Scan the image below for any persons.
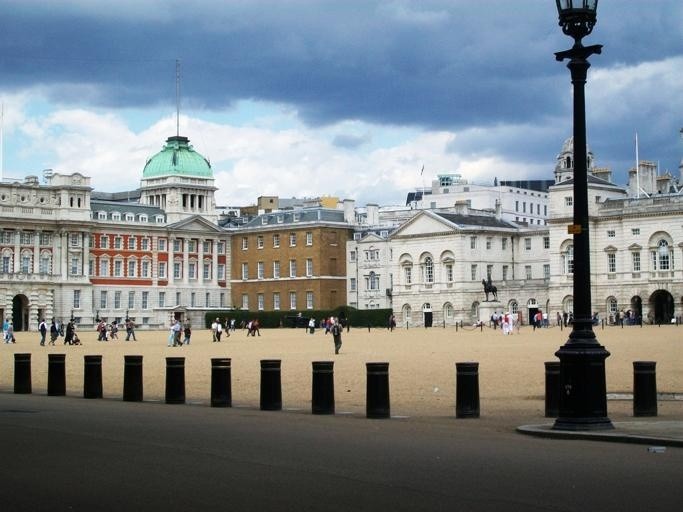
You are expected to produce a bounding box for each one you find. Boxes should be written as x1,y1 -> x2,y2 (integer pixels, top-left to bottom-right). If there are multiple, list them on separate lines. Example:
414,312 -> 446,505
492,312 -> 521,335
389,315 -> 395,330
533,311 -> 548,329
97,319 -> 137,341
477,320 -> 480,327
167,318 -> 192,347
557,310 -> 635,328
2,317 -> 75,346
211,317 -> 261,342
309,317 -> 345,354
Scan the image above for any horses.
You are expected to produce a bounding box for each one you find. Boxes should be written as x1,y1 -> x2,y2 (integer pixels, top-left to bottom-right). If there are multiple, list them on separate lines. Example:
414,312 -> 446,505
481,279 -> 498,301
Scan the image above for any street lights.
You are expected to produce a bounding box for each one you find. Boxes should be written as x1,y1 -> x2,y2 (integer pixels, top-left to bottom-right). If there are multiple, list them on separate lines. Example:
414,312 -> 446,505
551,0 -> 615,432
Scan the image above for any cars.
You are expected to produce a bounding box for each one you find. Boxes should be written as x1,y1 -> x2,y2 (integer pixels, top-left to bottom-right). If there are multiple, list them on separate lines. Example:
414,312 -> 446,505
282,316 -> 320,329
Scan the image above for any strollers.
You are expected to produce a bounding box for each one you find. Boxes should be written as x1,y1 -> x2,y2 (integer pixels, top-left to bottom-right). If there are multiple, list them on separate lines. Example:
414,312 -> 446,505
70,331 -> 82,346
